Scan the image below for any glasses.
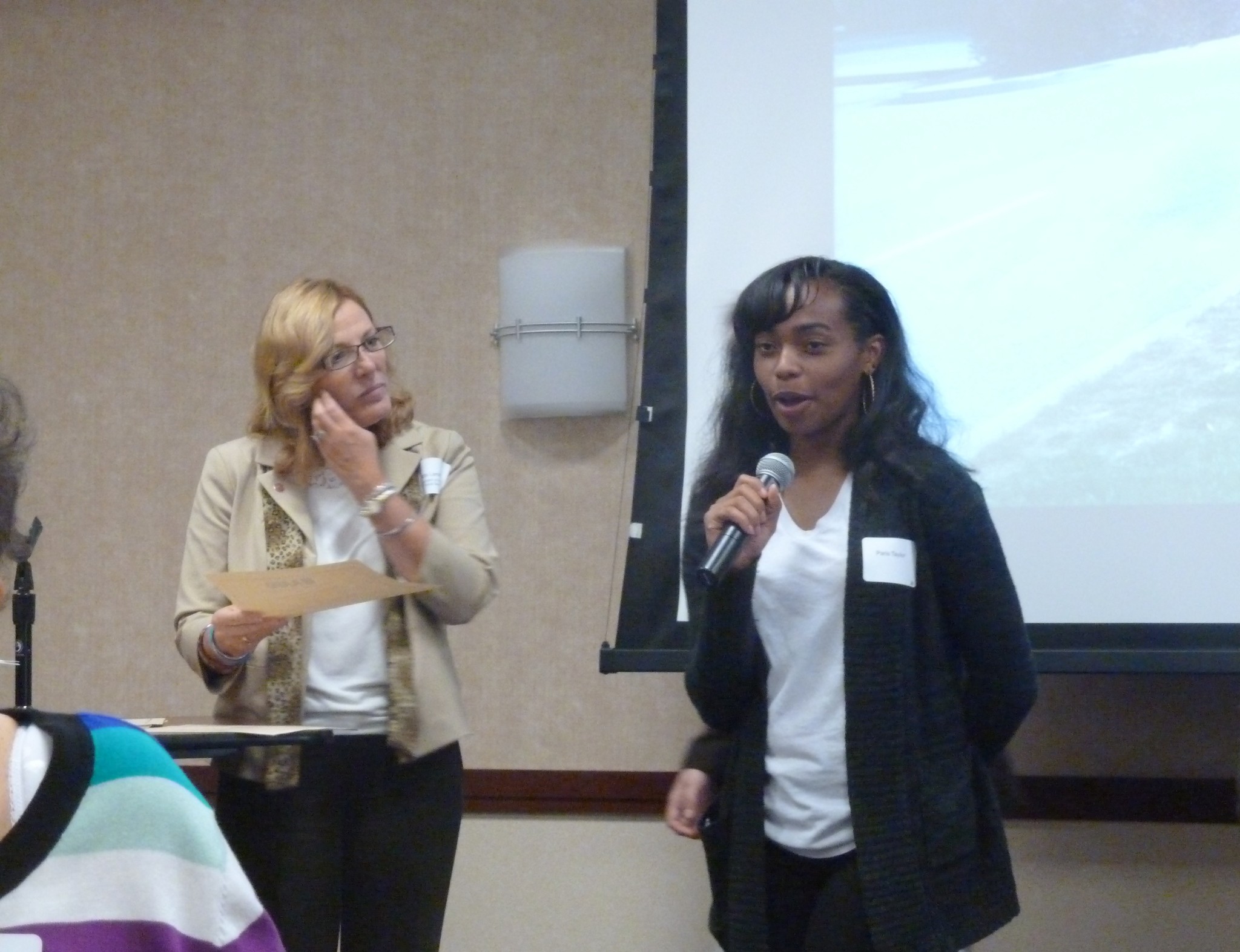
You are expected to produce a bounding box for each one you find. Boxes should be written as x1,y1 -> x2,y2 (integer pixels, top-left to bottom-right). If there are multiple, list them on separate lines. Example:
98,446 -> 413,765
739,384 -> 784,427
317,326 -> 395,371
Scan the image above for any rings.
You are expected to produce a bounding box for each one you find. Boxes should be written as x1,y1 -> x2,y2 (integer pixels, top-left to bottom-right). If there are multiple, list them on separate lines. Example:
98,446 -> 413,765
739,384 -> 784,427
311,427 -> 327,443
242,635 -> 252,642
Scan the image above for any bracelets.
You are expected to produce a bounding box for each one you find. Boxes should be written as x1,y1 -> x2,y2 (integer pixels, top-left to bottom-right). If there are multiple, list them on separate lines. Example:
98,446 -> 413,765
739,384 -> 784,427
203,624 -> 250,665
375,511 -> 420,538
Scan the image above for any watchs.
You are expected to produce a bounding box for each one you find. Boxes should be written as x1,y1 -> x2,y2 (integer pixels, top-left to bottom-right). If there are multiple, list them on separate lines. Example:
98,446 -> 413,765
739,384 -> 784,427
358,482 -> 398,517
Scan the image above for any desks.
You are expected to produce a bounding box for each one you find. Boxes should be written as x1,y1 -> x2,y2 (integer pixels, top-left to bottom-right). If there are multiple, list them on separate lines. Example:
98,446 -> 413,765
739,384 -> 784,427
128,726 -> 398,952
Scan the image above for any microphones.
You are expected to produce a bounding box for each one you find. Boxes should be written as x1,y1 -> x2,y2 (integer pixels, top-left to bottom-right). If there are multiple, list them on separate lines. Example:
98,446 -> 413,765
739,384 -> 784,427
695,452 -> 794,588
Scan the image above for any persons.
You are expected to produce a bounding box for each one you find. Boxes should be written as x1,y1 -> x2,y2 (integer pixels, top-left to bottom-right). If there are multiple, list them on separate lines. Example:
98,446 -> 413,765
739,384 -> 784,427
0,377 -> 287,952
172,276 -> 500,952
666,723 -> 718,838
681,256 -> 1037,952
